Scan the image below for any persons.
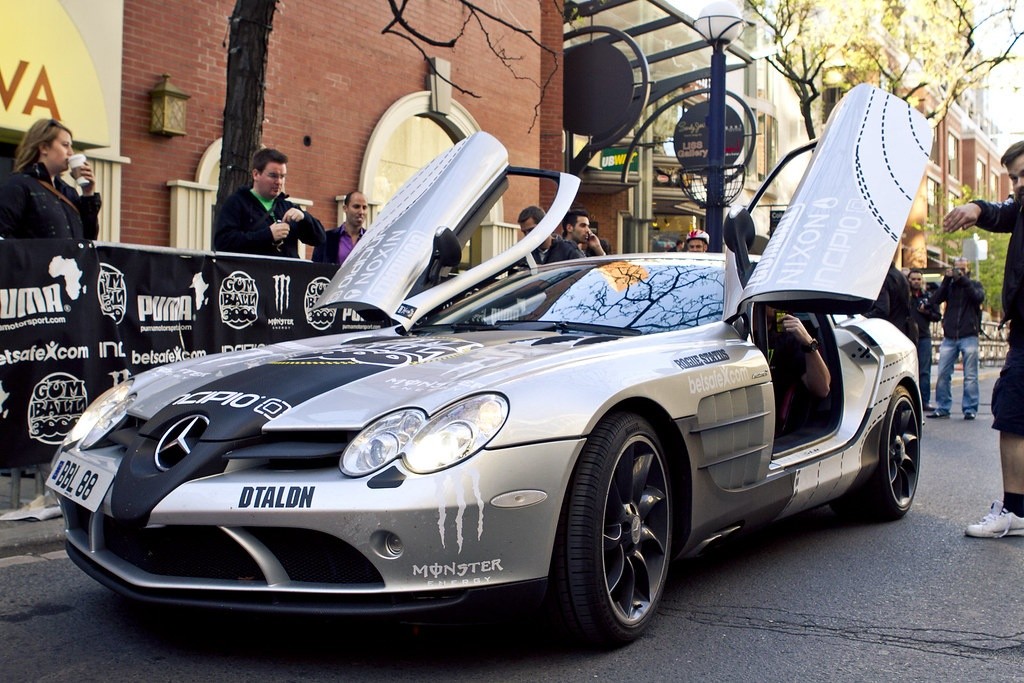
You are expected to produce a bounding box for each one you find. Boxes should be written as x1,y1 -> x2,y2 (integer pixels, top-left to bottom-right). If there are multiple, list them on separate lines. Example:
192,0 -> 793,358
560,210 -> 609,257
685,229 -> 711,251
0,119 -> 103,243
312,192 -> 367,264
667,240 -> 685,252
942,140 -> 1024,538
907,270 -> 943,410
765,305 -> 830,399
212,147 -> 327,258
514,205 -> 587,272
920,257 -> 985,420
863,247 -> 919,345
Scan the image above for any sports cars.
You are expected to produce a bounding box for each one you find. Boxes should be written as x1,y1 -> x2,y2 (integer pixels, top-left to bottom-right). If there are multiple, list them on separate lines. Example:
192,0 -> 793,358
42,83 -> 936,649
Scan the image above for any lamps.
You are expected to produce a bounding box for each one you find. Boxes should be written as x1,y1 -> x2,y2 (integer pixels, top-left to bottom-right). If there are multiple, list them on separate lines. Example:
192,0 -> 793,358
149,72 -> 189,136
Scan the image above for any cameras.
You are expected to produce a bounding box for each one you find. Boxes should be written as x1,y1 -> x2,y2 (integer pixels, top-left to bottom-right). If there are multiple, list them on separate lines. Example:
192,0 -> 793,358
952,267 -> 965,275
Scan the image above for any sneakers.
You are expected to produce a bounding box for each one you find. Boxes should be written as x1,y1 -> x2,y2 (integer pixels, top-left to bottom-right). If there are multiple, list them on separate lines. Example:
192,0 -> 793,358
965,500 -> 1024,538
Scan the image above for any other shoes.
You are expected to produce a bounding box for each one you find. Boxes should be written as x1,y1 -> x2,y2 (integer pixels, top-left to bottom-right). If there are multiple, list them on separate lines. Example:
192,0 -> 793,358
965,412 -> 974,419
926,412 -> 950,419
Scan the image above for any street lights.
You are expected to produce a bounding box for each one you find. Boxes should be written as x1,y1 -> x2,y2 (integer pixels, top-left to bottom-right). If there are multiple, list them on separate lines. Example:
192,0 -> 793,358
694,1 -> 749,252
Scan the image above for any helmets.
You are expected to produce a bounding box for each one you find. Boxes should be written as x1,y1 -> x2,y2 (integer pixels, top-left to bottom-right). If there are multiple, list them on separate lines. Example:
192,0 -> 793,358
686,229 -> 710,245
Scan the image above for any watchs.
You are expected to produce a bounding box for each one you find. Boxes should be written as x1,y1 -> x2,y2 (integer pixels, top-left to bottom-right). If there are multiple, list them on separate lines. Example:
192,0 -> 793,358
804,338 -> 818,353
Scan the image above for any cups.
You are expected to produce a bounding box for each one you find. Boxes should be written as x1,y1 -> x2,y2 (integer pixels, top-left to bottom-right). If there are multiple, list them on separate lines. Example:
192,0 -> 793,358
67,154 -> 89,186
